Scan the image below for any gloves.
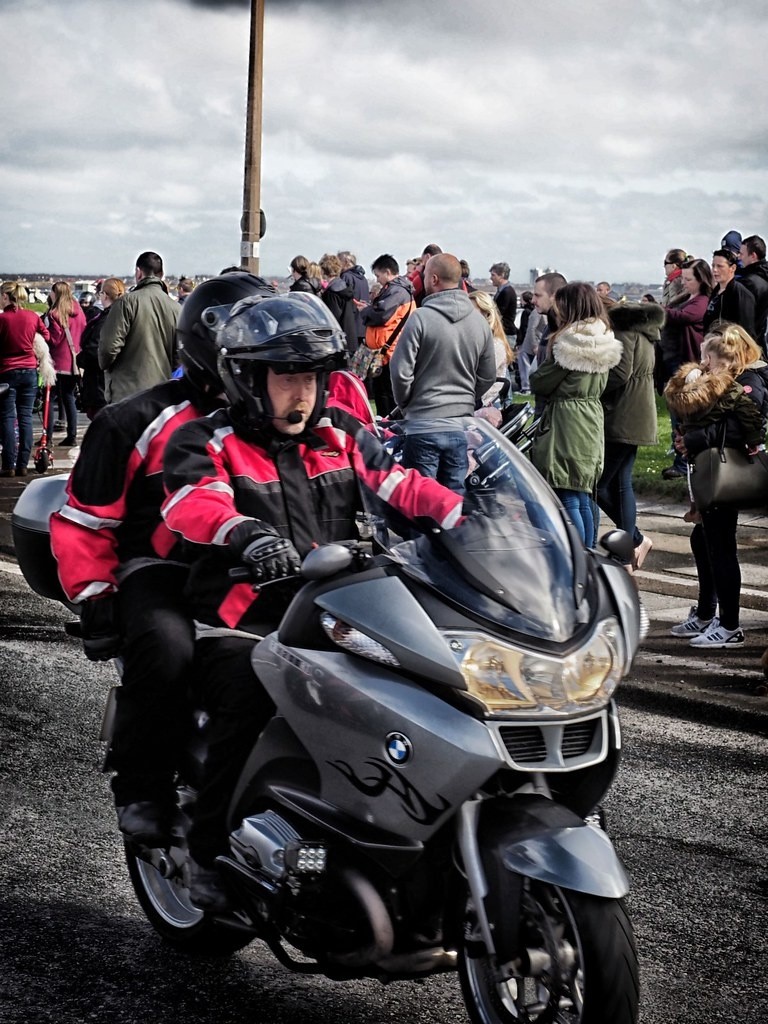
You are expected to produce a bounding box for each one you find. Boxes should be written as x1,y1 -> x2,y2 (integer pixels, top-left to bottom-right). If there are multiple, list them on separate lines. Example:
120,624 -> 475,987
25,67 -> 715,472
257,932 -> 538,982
228,520 -> 303,583
81,594 -> 133,663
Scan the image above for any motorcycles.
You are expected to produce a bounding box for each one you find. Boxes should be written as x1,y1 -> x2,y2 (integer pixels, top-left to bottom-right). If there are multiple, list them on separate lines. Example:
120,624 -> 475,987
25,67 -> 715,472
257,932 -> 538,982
12,409 -> 646,1024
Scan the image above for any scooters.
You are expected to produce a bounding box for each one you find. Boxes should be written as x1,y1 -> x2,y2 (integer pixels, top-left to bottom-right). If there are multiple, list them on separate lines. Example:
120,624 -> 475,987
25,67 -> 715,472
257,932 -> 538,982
32,366 -> 56,475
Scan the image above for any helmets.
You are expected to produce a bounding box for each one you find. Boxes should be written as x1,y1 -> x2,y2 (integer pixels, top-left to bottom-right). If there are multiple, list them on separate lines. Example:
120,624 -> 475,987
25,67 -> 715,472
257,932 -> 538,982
176,270 -> 281,397
218,292 -> 350,430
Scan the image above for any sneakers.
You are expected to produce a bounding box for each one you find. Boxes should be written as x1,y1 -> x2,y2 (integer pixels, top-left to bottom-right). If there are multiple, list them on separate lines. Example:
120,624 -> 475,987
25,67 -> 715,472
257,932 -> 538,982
671,605 -> 716,637
689,616 -> 744,648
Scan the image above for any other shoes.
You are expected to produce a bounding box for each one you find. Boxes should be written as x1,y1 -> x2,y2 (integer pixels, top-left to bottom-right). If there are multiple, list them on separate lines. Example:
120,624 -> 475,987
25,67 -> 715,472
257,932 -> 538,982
661,465 -> 686,479
15,467 -> 27,476
35,438 -> 52,446
631,537 -> 654,568
182,856 -> 226,910
58,434 -> 77,447
117,801 -> 175,839
0,469 -> 16,478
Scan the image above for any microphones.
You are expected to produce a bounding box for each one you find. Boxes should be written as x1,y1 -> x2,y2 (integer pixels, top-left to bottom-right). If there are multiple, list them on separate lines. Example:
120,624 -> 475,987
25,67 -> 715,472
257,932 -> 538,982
259,412 -> 303,424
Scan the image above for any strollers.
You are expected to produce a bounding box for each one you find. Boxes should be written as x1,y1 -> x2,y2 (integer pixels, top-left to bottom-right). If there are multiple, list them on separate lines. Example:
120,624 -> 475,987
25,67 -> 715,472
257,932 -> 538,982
328,369 -> 550,491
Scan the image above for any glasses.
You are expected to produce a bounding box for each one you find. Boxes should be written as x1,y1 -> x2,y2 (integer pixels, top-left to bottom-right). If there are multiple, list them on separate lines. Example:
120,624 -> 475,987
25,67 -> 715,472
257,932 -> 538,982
664,259 -> 679,266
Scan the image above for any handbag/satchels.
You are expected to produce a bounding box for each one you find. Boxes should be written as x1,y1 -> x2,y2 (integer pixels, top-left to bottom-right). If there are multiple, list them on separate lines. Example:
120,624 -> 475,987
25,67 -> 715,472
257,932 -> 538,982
350,343 -> 383,382
73,352 -> 83,375
689,423 -> 768,514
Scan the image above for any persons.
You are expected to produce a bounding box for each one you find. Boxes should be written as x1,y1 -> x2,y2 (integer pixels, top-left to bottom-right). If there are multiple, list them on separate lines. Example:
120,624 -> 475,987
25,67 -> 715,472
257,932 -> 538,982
51,268 -> 276,848
0,229 -> 768,650
153,292 -> 475,867
